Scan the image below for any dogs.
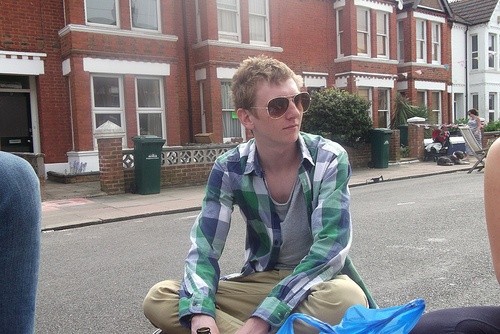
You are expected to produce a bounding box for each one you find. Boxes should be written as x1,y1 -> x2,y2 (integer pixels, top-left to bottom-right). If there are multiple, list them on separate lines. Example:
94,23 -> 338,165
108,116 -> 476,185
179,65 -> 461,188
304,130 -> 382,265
436,150 -> 470,166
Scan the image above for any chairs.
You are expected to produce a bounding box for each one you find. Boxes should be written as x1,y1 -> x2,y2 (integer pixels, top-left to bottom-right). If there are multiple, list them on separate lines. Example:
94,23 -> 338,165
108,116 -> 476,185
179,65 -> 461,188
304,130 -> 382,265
459,126 -> 489,174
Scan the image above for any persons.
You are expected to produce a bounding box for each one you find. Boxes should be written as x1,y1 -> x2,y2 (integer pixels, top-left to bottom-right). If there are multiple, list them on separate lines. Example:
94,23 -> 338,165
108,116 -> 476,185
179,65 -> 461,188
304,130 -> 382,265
143,56 -> 380,334
432,126 -> 452,156
462,109 -> 481,161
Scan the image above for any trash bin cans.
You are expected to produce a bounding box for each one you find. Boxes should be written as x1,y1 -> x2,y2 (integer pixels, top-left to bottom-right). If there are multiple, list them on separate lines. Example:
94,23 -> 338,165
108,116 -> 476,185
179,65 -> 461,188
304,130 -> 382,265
367,128 -> 392,168
130,135 -> 166,194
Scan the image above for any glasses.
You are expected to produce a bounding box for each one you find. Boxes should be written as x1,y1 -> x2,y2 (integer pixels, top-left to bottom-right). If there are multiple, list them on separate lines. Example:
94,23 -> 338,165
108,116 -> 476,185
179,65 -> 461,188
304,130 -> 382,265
247,92 -> 311,119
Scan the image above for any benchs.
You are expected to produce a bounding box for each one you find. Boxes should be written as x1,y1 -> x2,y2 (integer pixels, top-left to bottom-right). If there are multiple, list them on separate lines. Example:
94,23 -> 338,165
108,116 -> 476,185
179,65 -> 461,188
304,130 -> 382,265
424,146 -> 449,162
